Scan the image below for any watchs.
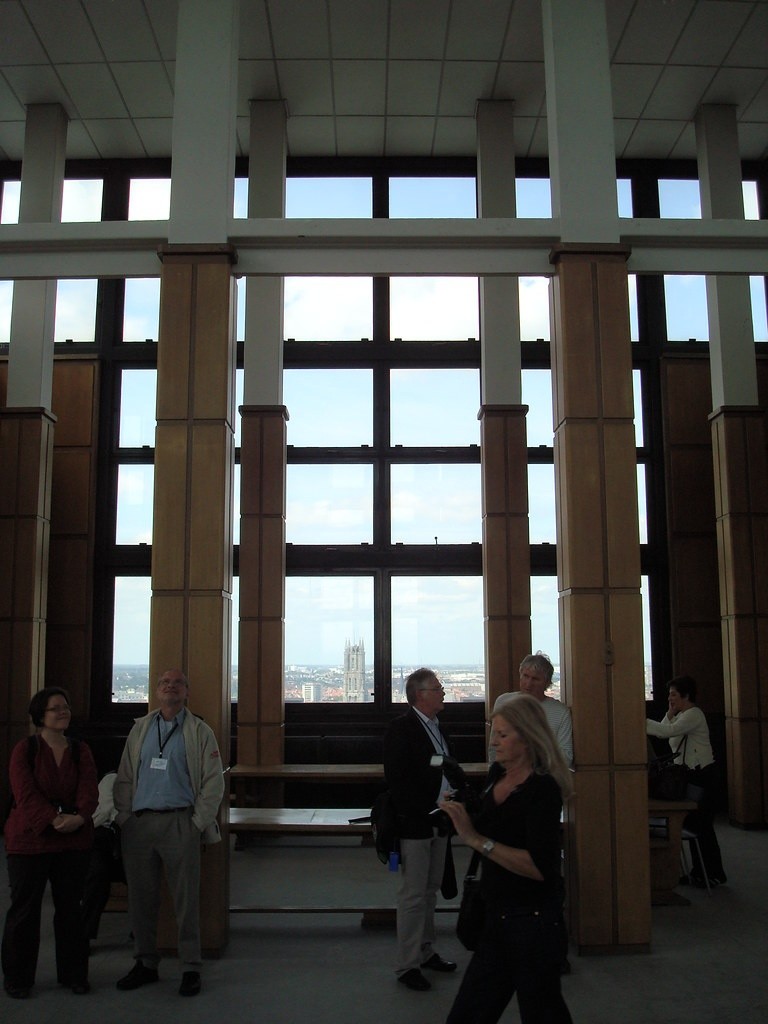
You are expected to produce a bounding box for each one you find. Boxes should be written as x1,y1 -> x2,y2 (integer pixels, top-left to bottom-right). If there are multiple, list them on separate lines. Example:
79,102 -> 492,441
482,840 -> 496,856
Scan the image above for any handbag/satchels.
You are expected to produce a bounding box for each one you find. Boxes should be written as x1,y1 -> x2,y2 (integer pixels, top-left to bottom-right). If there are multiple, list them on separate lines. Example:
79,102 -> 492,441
441,836 -> 458,900
456,877 -> 489,951
648,763 -> 690,801
370,791 -> 402,871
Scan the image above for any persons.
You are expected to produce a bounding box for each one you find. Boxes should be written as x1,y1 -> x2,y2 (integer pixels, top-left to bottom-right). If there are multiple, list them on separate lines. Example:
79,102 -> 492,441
647,677 -> 727,888
383,669 -> 467,991
488,655 -> 572,975
0,686 -> 99,999
80,773 -> 135,952
439,694 -> 574,1024
113,671 -> 225,995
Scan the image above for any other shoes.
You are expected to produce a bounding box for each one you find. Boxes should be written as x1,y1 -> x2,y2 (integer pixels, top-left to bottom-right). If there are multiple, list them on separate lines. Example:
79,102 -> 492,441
178,971 -> 200,996
560,958 -> 572,974
420,953 -> 457,972
116,960 -> 158,990
70,982 -> 91,995
679,874 -> 727,889
398,969 -> 431,991
3,981 -> 30,999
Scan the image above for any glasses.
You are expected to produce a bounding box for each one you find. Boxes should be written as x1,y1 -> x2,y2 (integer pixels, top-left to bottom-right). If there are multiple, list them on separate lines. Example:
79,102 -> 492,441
419,687 -> 444,694
157,680 -> 188,687
45,704 -> 71,713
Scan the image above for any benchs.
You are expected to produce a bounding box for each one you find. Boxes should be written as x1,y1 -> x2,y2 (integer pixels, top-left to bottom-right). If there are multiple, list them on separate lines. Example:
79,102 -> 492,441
228,806 -> 564,931
230,763 -> 493,852
648,799 -> 700,906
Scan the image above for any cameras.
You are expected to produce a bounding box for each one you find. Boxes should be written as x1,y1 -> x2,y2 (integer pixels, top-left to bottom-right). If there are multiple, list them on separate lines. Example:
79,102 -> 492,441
429,753 -> 489,840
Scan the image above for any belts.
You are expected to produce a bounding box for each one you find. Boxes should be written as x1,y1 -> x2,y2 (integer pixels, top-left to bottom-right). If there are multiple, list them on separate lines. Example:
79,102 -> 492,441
132,807 -> 187,817
